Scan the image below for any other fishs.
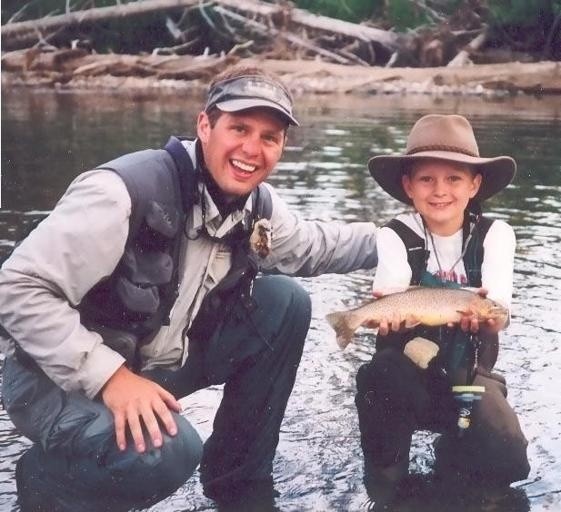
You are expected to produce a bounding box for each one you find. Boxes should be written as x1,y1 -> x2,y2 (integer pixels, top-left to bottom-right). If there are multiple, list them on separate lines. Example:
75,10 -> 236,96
324,286 -> 510,351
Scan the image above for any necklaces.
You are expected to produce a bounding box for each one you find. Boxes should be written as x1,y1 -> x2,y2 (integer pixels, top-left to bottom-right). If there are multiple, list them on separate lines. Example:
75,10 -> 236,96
419,212 -> 479,288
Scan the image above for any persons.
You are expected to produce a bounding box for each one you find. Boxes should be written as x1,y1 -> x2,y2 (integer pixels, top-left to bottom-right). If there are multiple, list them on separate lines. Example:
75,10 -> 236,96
355,115 -> 530,512
0,69 -> 377,512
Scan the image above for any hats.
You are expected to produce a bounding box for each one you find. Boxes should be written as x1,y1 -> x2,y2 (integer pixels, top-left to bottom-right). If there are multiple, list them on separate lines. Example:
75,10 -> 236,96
204,75 -> 300,126
368,113 -> 518,206
